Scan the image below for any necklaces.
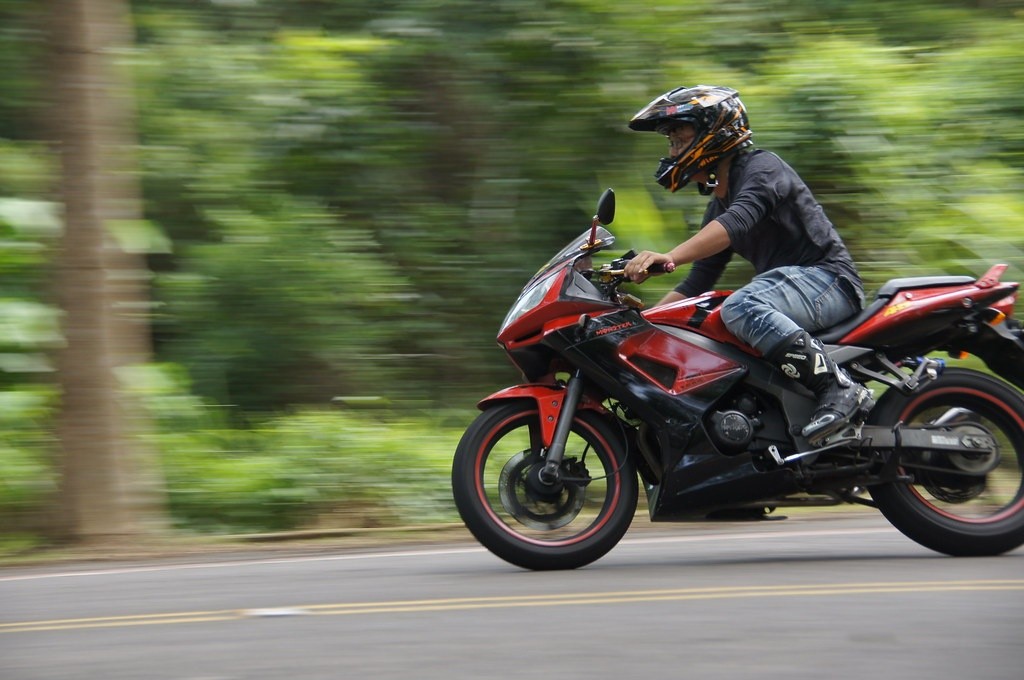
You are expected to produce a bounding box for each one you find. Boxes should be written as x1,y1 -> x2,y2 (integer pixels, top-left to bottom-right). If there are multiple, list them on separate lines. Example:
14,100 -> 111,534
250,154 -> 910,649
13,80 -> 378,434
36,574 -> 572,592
714,171 -> 730,210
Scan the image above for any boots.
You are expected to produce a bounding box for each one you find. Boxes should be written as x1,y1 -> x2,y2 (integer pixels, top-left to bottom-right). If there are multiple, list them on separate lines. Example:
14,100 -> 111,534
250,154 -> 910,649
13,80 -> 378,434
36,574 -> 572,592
761,328 -> 870,445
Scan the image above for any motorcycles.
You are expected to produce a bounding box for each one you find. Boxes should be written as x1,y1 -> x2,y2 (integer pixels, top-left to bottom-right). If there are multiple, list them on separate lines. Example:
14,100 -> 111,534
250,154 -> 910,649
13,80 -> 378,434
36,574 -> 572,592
450,188 -> 1024,574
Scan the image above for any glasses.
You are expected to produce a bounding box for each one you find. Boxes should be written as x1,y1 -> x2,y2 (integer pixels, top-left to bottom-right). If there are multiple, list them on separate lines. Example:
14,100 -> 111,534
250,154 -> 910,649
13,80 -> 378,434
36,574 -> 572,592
663,133 -> 695,149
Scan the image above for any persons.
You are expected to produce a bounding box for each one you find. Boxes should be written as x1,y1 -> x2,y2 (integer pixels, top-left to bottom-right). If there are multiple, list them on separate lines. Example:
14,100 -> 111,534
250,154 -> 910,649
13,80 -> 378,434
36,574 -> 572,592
624,86 -> 868,438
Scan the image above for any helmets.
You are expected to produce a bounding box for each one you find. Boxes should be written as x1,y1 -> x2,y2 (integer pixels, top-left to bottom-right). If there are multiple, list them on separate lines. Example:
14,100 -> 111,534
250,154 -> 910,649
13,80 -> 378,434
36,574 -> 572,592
628,85 -> 753,194
